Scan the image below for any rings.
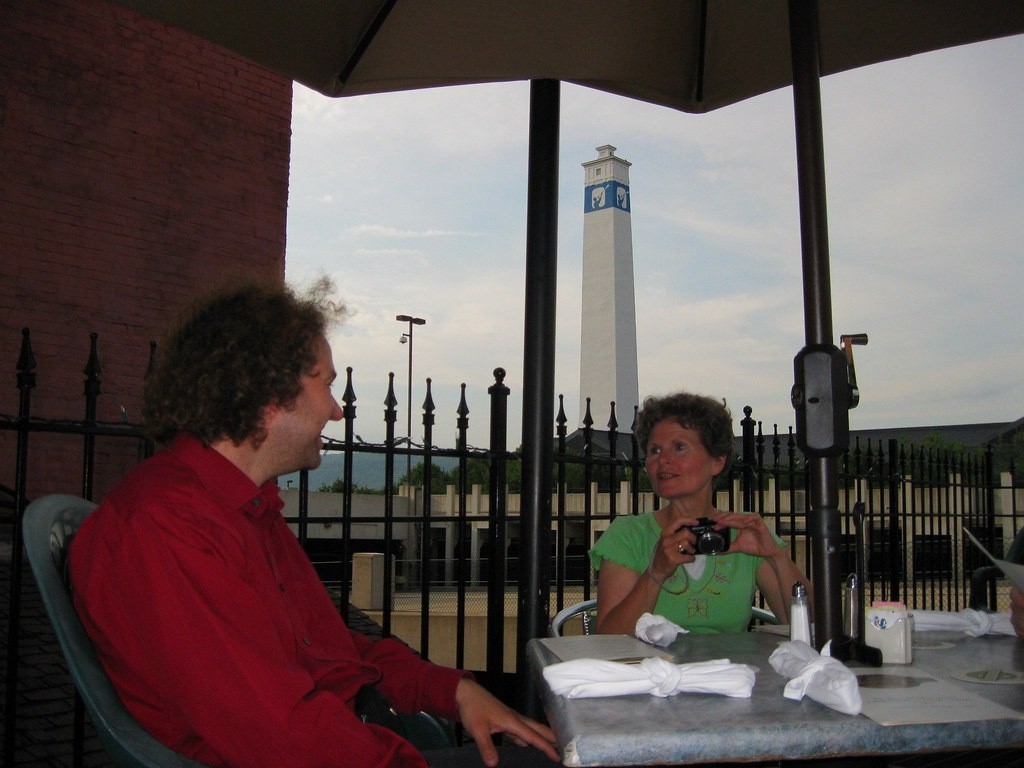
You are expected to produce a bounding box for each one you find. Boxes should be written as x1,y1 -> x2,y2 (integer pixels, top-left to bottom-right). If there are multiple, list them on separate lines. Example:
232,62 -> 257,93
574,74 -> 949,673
675,541 -> 686,553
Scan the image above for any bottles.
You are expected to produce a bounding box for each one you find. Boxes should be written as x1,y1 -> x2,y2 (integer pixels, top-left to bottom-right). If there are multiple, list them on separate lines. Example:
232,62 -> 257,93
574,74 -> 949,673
789,580 -> 811,647
843,571 -> 859,639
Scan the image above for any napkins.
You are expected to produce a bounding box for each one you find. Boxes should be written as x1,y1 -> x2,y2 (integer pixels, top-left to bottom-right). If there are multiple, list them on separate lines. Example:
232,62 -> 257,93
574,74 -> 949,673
633,612 -> 690,647
542,655 -> 756,701
767,638 -> 863,717
910,608 -> 1016,637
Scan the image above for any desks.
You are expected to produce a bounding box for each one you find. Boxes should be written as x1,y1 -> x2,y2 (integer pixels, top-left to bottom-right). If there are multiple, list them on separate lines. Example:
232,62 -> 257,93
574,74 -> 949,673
525,631 -> 1024,768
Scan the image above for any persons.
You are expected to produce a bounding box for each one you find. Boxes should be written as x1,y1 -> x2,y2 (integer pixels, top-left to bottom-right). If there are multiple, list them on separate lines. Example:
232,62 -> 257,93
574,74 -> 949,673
587,393 -> 814,636
67,280 -> 561,768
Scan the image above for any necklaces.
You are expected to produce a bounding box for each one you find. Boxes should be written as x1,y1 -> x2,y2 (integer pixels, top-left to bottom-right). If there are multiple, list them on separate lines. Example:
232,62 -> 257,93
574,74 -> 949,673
676,555 -> 717,594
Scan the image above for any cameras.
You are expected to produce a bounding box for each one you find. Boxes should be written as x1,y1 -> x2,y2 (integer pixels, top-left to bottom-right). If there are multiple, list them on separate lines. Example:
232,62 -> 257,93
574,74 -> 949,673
677,518 -> 730,556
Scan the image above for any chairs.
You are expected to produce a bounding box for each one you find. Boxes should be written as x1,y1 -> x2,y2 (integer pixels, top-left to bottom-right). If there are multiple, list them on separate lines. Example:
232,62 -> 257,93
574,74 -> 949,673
548,599 -> 780,638
22,493 -> 462,768
